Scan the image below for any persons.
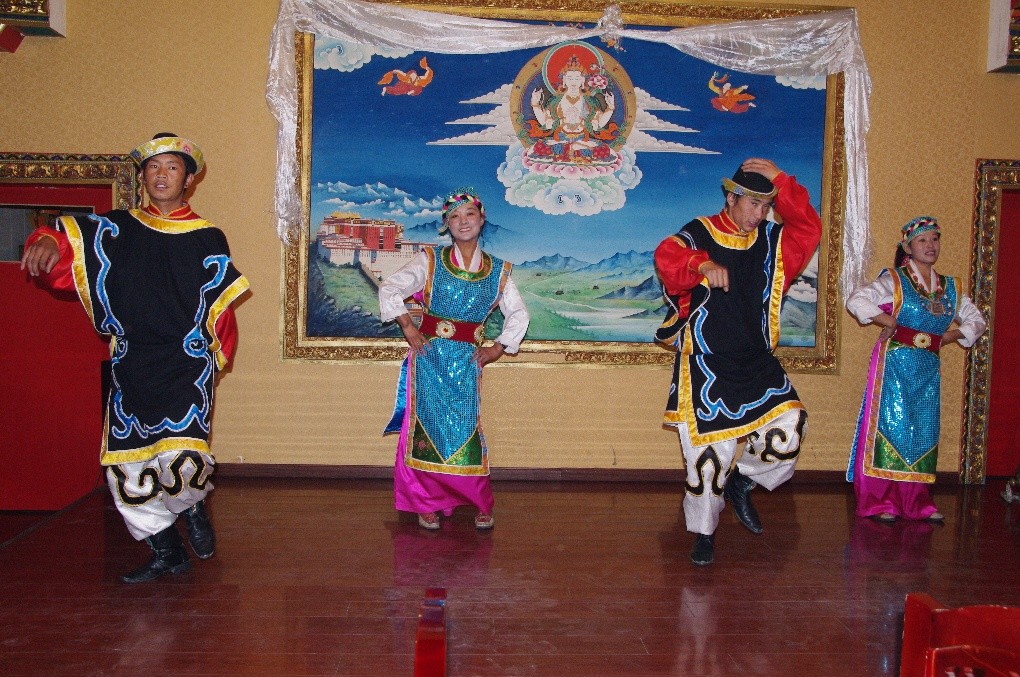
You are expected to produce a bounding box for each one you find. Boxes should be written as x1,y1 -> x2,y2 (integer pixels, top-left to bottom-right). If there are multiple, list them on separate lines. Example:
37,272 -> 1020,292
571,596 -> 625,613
654,159 -> 822,569
376,186 -> 532,532
18,133 -> 255,584
841,218 -> 989,523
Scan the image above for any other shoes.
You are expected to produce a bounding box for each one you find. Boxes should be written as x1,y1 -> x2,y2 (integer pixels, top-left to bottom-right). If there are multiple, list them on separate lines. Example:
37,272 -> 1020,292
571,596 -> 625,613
873,513 -> 896,522
418,512 -> 440,529
926,511 -> 945,521
474,507 -> 493,529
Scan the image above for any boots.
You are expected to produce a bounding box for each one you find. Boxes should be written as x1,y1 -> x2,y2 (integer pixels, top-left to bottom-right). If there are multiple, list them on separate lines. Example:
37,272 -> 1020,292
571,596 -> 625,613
724,465 -> 763,534
185,500 -> 214,559
691,534 -> 714,565
120,519 -> 190,582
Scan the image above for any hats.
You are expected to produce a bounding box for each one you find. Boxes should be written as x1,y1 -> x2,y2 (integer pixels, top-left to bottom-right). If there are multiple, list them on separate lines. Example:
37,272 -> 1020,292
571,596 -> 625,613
902,216 -> 941,246
129,133 -> 204,176
722,163 -> 778,198
438,187 -> 486,233
